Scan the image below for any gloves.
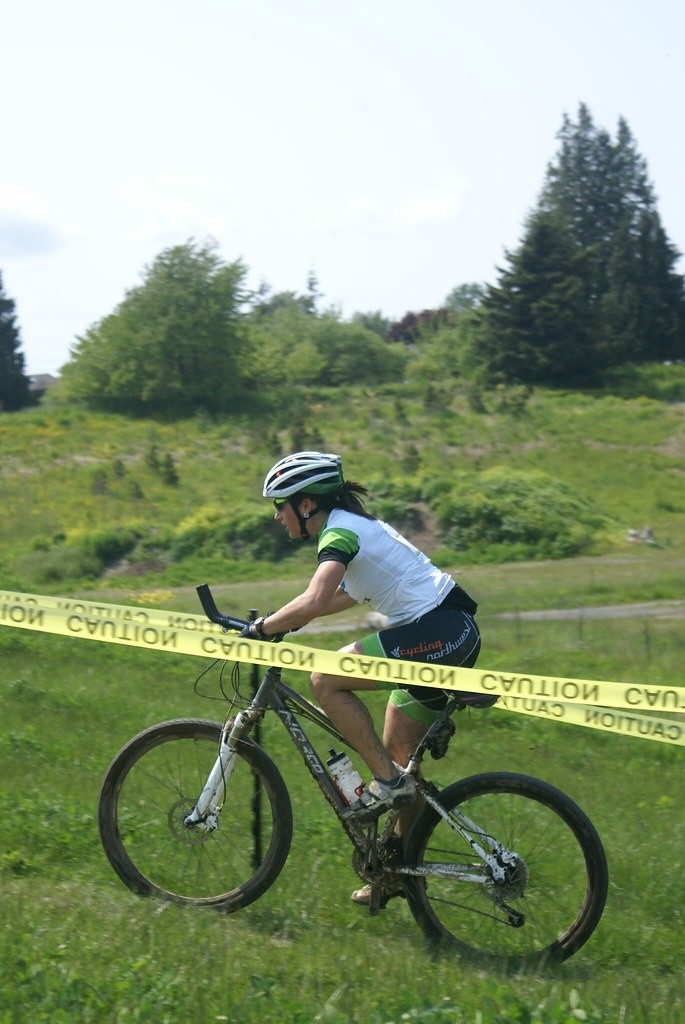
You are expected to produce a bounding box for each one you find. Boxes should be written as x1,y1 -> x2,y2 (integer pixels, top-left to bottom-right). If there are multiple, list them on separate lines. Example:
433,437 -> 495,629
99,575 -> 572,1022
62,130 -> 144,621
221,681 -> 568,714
267,612 -> 300,634
237,621 -> 263,641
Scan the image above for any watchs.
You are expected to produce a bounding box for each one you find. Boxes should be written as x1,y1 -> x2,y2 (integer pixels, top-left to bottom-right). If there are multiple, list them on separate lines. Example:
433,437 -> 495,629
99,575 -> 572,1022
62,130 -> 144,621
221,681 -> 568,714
254,615 -> 270,639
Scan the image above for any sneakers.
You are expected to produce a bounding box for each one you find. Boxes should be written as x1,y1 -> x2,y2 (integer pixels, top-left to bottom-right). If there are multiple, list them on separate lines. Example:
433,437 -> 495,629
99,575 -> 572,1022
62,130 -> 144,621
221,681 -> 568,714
339,773 -> 417,824
351,880 -> 402,904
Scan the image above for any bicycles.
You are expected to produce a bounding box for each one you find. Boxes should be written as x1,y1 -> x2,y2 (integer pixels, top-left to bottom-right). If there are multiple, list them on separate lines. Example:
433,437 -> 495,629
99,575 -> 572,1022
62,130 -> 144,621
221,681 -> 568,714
96,579 -> 609,974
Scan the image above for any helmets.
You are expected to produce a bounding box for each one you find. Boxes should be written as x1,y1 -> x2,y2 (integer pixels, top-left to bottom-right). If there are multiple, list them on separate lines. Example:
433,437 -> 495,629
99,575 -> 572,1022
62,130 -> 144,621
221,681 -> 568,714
263,451 -> 344,497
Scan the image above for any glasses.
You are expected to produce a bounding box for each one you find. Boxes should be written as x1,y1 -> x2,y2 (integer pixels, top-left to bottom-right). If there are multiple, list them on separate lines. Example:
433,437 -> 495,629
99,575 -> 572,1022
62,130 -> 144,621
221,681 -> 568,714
272,499 -> 288,511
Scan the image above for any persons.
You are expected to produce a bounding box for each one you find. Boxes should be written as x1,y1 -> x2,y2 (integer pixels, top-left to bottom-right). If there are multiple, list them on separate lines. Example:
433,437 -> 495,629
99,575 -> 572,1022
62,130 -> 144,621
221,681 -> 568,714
242,451 -> 482,904
